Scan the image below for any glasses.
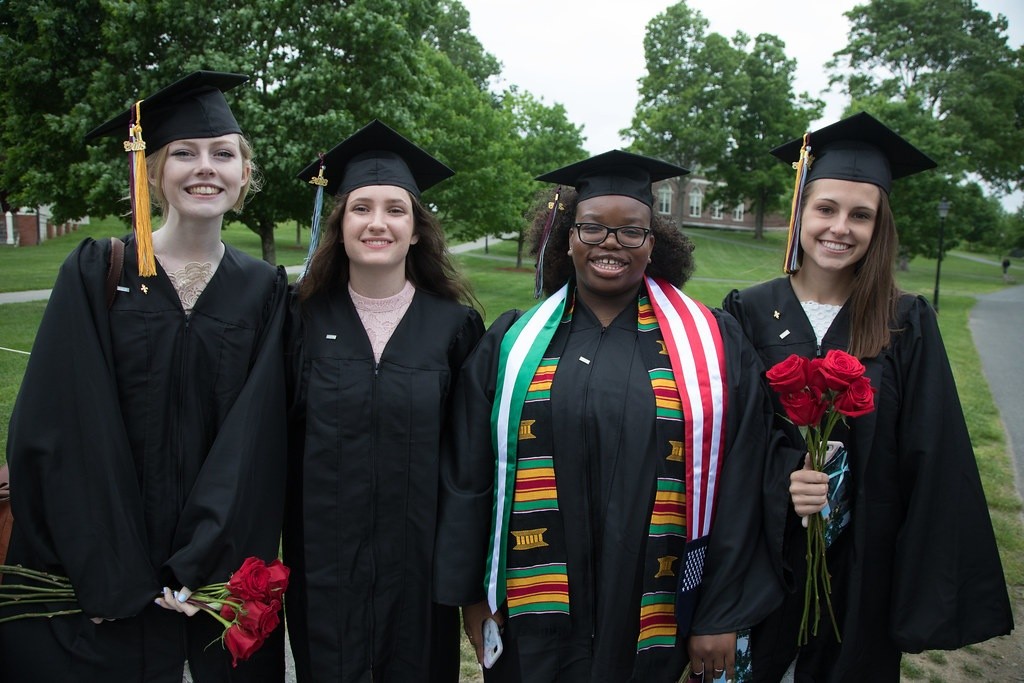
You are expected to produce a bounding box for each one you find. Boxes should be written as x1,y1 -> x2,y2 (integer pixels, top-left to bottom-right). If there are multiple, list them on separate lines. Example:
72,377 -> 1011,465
574,222 -> 650,248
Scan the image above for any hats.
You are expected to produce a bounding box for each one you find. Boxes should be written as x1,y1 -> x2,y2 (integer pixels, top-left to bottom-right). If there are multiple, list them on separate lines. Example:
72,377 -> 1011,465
82,69 -> 250,277
770,111 -> 938,276
533,148 -> 692,300
296,118 -> 455,285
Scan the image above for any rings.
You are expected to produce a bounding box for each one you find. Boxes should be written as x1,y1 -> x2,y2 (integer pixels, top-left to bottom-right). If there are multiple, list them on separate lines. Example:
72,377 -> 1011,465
694,671 -> 702,675
470,636 -> 472,638
715,669 -> 723,672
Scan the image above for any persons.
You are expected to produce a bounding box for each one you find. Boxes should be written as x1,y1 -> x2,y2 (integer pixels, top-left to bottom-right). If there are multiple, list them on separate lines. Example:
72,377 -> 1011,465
1002,258 -> 1010,273
722,110 -> 1014,683
282,119 -> 486,683
434,149 -> 792,683
0,70 -> 288,683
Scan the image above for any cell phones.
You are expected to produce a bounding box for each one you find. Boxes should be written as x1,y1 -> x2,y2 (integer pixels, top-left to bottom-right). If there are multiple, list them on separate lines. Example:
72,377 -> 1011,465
482,618 -> 503,669
